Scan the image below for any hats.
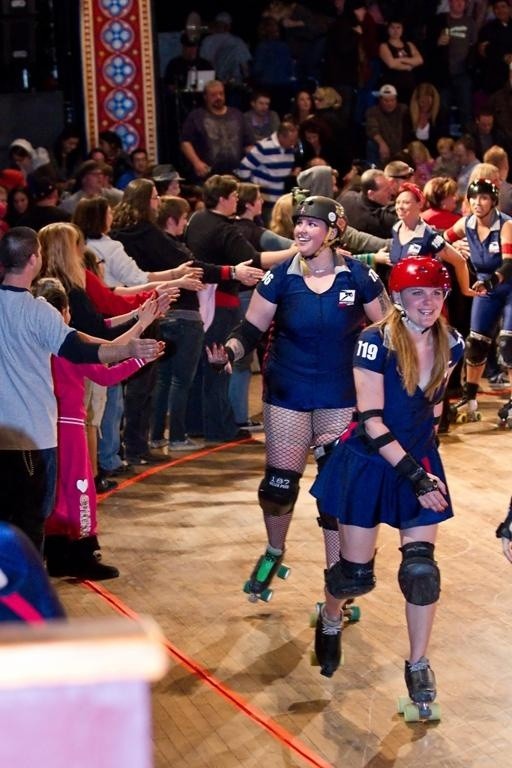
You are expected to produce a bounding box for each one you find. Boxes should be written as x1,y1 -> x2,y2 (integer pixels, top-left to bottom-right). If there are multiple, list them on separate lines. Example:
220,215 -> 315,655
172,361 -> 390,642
8,137 -> 36,159
150,163 -> 186,184
378,84 -> 399,96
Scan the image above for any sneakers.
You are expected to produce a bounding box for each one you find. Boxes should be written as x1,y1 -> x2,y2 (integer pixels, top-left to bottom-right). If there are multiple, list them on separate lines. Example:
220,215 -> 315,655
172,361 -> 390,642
96,419 -> 263,494
489,376 -> 511,388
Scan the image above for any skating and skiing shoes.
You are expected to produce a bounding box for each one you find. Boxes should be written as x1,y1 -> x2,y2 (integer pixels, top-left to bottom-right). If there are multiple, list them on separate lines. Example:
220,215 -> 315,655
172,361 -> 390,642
449,395 -> 481,424
397,658 -> 443,725
242,542 -> 292,603
498,398 -> 512,430
308,598 -> 361,677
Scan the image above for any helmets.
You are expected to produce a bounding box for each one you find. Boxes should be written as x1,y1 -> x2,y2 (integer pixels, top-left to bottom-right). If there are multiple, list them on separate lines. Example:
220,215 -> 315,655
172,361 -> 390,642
292,197 -> 347,242
389,255 -> 451,294
467,179 -> 500,209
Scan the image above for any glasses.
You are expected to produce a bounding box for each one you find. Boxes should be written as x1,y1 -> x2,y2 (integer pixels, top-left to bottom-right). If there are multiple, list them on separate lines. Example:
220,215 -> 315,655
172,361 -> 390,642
388,167 -> 415,180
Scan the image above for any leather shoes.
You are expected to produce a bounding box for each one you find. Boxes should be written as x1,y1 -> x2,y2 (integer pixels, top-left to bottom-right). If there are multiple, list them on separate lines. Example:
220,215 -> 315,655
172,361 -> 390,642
45,552 -> 119,582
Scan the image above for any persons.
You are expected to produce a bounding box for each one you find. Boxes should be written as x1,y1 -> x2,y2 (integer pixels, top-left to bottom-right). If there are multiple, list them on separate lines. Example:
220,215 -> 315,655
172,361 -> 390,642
306,254 -> 465,724
202,195 -> 394,604
1,0 -> 512,582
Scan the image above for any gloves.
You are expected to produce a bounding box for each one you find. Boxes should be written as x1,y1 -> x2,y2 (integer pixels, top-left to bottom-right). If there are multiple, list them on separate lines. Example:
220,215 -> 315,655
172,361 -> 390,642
206,346 -> 236,375
480,273 -> 500,292
393,452 -> 440,499
